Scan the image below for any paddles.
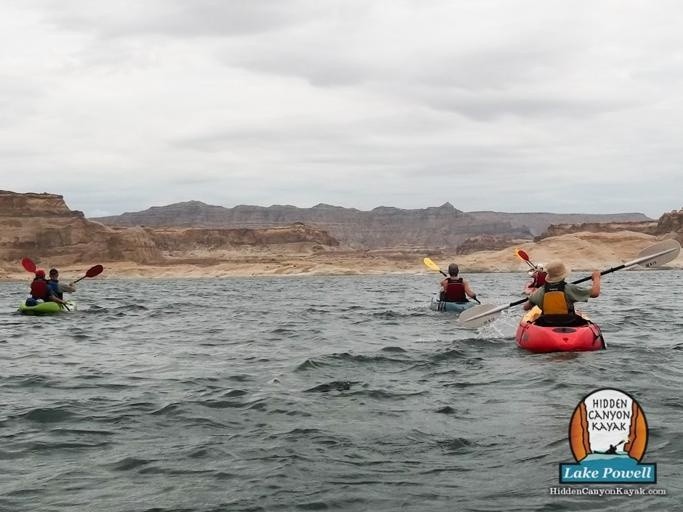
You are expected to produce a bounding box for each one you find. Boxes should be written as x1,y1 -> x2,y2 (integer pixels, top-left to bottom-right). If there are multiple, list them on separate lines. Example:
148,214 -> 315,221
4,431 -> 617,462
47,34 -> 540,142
68,264 -> 103,285
459,239 -> 681,326
424,257 -> 481,305
516,249 -> 537,269
21,258 -> 71,312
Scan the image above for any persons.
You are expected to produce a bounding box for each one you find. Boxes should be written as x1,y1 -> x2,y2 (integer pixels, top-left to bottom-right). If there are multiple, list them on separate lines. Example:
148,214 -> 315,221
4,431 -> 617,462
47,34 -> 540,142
440,264 -> 477,303
607,444 -> 617,452
31,270 -> 66,304
46,268 -> 77,300
524,261 -> 602,327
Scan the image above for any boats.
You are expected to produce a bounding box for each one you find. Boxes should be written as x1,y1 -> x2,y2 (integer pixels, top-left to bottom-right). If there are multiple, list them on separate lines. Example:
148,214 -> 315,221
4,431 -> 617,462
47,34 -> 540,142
431,294 -> 481,312
514,314 -> 606,353
19,301 -> 66,314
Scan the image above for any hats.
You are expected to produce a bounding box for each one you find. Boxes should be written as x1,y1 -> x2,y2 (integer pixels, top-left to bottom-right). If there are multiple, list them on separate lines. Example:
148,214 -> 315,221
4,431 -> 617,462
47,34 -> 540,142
449,263 -> 458,274
36,270 -> 45,277
545,259 -> 570,283
